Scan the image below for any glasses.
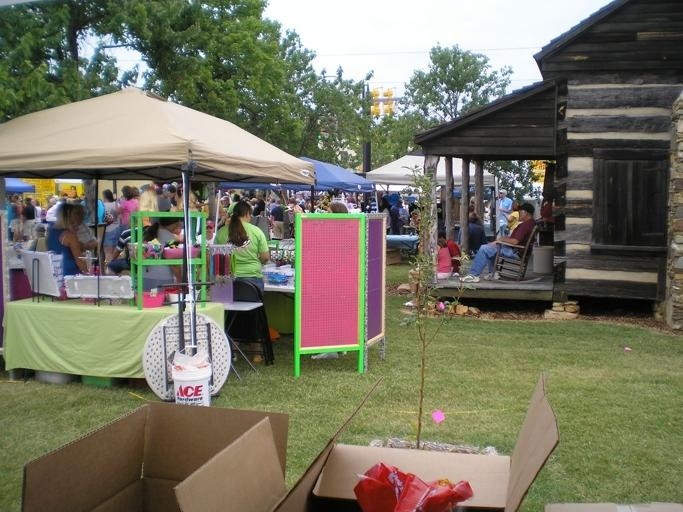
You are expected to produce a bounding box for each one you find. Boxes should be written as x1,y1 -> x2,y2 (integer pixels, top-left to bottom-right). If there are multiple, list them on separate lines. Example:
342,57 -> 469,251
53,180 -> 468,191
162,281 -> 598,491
206,226 -> 213,229
500,192 -> 504,195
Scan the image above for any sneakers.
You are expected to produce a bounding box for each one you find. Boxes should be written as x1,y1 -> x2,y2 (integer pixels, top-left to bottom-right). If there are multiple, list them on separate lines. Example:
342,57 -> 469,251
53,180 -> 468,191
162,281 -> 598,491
253,353 -> 264,362
459,274 -> 480,283
483,272 -> 503,281
338,351 -> 346,357
232,354 -> 236,361
311,353 -> 339,360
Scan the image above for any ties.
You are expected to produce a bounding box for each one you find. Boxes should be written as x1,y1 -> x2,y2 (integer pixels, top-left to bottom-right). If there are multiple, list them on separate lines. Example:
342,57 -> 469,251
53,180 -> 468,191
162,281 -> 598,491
499,200 -> 503,208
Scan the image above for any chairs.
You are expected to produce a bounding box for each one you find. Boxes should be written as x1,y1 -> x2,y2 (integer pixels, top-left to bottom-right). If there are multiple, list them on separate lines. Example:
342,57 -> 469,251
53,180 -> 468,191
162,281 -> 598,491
491,224 -> 539,281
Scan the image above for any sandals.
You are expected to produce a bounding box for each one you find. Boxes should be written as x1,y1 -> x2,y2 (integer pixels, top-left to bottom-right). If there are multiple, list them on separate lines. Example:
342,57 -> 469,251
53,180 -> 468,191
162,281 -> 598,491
402,302 -> 416,307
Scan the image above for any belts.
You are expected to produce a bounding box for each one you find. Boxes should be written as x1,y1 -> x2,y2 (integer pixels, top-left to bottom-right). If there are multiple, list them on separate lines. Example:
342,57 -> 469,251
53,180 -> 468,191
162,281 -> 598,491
88,225 -> 105,227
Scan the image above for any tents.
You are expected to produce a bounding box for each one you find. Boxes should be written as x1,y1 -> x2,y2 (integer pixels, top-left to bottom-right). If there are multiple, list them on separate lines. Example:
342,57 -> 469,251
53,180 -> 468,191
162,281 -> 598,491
0,87 -> 316,351
217,155 -> 374,187
365,154 -> 506,185
1,178 -> 37,191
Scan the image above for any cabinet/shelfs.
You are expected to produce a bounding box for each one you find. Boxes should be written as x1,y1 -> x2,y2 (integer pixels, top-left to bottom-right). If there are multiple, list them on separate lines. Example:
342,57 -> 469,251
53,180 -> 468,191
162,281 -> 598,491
130,211 -> 207,311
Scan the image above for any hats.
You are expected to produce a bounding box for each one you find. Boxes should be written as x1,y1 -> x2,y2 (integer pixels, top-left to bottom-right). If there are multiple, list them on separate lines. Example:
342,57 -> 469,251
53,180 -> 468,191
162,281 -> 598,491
143,216 -> 151,228
35,224 -> 46,231
206,220 -> 214,227
469,212 -> 480,219
220,196 -> 229,200
513,203 -> 535,214
499,189 -> 507,195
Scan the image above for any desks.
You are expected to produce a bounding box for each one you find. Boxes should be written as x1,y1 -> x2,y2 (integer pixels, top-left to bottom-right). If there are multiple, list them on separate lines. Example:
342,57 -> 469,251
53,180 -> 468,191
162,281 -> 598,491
5,292 -> 264,383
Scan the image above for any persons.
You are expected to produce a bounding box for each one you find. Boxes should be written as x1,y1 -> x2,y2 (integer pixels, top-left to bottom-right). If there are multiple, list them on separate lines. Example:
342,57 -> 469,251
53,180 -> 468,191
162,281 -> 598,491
106,214 -> 142,273
469,195 -> 477,212
42,203 -> 93,277
216,189 -> 276,200
431,238 -> 452,281
439,232 -> 459,277
4,195 -> 21,244
250,213 -> 277,241
277,188 -> 316,237
22,197 -> 38,243
495,188 -> 512,238
501,203 -> 518,237
119,186 -> 137,226
213,200 -> 276,365
458,205 -> 489,253
317,191 -> 420,236
101,189 -> 119,262
459,203 -> 532,282
483,200 -> 491,218
139,182 -> 209,215
79,194 -> 103,258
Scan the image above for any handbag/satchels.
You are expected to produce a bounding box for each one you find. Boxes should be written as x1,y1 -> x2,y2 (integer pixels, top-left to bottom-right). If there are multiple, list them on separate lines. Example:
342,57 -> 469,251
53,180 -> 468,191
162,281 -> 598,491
505,220 -> 517,234
104,212 -> 113,224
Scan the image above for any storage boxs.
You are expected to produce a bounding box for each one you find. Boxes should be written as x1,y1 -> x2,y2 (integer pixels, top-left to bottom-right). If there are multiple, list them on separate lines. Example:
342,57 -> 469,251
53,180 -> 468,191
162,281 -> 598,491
20,400 -> 290,512
271,372 -> 560,512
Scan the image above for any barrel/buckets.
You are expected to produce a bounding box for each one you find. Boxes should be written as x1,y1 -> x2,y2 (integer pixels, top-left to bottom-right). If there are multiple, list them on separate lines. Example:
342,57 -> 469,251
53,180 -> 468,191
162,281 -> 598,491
533,246 -> 555,273
171,345 -> 213,407
